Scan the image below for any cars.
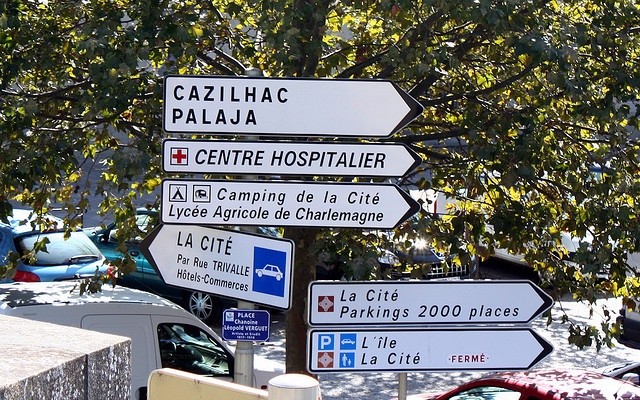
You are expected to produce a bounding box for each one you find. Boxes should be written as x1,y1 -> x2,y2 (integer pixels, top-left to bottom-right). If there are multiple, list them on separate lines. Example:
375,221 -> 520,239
256,211 -> 479,281
389,368 -> 640,400
79,204 -> 282,323
433,185 -> 611,287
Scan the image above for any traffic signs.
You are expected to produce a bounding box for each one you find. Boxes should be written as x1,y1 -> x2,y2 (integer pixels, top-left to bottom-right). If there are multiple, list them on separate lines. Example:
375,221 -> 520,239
137,221 -> 296,312
160,178 -> 422,230
304,326 -> 555,375
307,278 -> 555,328
162,139 -> 422,178
162,75 -> 426,140
224,310 -> 271,341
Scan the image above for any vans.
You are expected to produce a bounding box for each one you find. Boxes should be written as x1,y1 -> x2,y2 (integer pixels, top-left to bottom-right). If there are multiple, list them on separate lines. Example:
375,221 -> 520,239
616,240 -> 640,349
0,282 -> 286,400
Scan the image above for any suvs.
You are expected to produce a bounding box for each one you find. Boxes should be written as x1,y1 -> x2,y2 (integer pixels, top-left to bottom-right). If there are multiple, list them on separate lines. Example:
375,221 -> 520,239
0,207 -> 120,284
